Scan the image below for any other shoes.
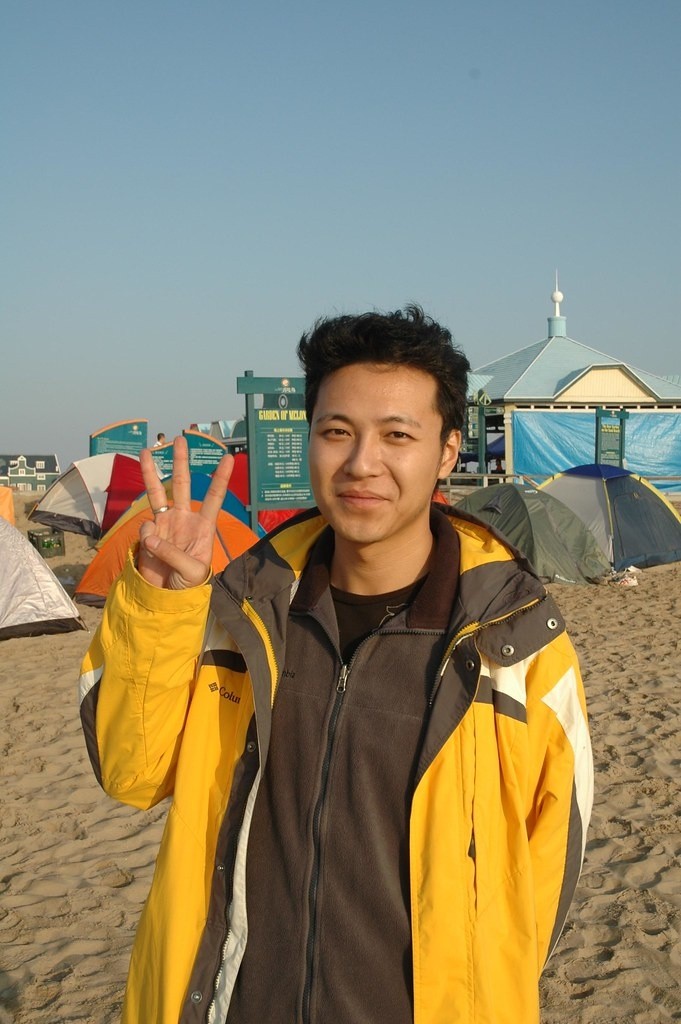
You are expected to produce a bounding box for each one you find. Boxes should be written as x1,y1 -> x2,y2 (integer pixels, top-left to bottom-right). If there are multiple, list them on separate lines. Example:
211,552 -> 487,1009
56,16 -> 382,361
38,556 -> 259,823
608,575 -> 639,587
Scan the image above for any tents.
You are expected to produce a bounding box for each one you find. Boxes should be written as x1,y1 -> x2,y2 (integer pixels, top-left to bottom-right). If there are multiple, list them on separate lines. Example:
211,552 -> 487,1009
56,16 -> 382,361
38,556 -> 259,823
1,452 -> 681,640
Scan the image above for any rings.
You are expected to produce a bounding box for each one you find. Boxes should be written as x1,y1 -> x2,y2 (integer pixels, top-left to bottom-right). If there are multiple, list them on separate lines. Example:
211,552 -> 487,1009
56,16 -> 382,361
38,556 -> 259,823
153,505 -> 170,513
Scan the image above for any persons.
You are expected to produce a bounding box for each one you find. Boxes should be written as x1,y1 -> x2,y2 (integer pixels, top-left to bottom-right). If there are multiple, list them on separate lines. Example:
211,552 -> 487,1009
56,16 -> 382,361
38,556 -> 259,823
80,305 -> 593,1024
153,432 -> 166,448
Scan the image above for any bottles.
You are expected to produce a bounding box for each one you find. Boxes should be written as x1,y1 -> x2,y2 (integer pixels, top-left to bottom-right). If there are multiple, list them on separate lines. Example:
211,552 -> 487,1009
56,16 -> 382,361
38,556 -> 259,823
30,530 -> 60,549
